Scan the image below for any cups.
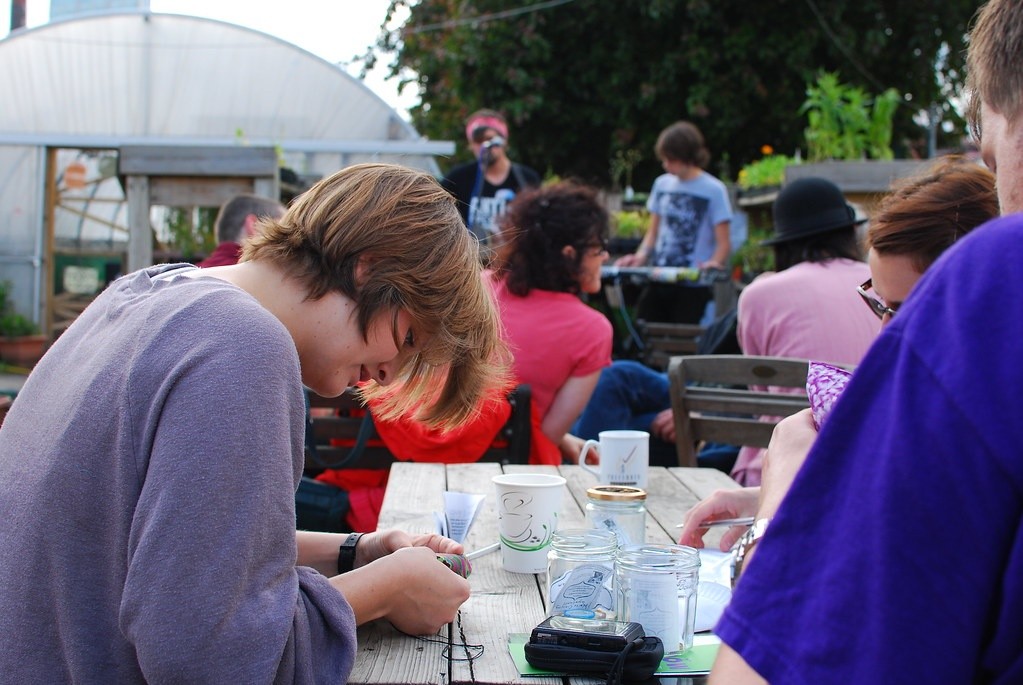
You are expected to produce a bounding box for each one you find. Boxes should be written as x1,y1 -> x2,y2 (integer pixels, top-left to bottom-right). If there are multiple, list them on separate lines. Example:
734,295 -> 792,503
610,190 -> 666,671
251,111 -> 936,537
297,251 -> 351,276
578,430 -> 649,488
493,475 -> 566,573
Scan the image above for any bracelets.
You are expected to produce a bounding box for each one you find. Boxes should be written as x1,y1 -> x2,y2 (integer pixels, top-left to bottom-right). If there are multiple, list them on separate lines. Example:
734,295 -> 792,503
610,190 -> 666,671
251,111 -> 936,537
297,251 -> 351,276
337,533 -> 364,574
731,516 -> 772,592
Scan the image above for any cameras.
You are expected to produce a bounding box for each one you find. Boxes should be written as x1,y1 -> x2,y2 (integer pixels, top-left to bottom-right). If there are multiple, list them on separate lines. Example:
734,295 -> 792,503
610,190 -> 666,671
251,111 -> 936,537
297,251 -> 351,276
529,615 -> 646,653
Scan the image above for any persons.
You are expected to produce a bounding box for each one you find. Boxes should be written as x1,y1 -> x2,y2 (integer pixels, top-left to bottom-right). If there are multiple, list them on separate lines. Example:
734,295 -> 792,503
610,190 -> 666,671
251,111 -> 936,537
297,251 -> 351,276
729,177 -> 887,486
1,162 -> 516,685
562,201 -> 871,465
706,0 -> 1023,685
614,121 -> 734,270
677,155 -> 1000,599
481,177 -> 613,465
438,109 -> 542,269
194,193 -> 287,268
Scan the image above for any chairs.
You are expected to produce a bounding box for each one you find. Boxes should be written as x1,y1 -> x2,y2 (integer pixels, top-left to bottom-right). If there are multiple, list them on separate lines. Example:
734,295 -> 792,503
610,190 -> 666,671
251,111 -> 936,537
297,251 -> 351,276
663,354 -> 857,471
631,320 -> 710,367
306,383 -> 533,469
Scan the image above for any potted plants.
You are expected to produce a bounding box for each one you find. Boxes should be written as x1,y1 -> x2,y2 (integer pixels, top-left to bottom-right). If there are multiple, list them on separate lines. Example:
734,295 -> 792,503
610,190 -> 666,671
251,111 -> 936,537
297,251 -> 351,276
0,279 -> 48,364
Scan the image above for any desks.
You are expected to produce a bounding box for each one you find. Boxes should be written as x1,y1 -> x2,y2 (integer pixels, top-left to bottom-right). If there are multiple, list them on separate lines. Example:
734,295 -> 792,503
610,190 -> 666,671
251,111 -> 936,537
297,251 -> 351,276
347,460 -> 744,685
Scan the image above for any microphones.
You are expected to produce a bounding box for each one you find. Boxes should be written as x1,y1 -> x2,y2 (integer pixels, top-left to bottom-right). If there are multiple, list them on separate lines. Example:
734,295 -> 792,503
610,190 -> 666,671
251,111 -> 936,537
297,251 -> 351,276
484,136 -> 504,148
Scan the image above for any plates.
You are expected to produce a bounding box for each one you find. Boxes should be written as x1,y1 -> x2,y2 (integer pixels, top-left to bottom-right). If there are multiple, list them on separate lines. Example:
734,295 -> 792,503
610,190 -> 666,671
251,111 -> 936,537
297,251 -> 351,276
690,578 -> 733,633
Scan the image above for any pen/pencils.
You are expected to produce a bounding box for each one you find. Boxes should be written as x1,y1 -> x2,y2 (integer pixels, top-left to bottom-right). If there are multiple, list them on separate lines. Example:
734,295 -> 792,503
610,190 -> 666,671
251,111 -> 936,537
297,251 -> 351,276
466,543 -> 500,562
676,517 -> 755,528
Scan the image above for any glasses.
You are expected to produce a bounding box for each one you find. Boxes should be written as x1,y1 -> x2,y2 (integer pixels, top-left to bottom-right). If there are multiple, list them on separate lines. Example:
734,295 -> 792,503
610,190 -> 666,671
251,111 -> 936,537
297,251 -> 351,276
856,277 -> 899,322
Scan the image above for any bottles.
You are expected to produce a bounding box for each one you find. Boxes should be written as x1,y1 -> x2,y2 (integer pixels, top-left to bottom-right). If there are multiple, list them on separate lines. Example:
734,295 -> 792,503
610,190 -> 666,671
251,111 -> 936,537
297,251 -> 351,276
585,487 -> 647,549
547,531 -> 618,621
614,543 -> 701,655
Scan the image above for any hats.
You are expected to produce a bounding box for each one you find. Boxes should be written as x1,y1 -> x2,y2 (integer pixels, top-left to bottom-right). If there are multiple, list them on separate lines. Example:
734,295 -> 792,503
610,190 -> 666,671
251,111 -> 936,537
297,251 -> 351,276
464,108 -> 509,141
760,176 -> 869,247
